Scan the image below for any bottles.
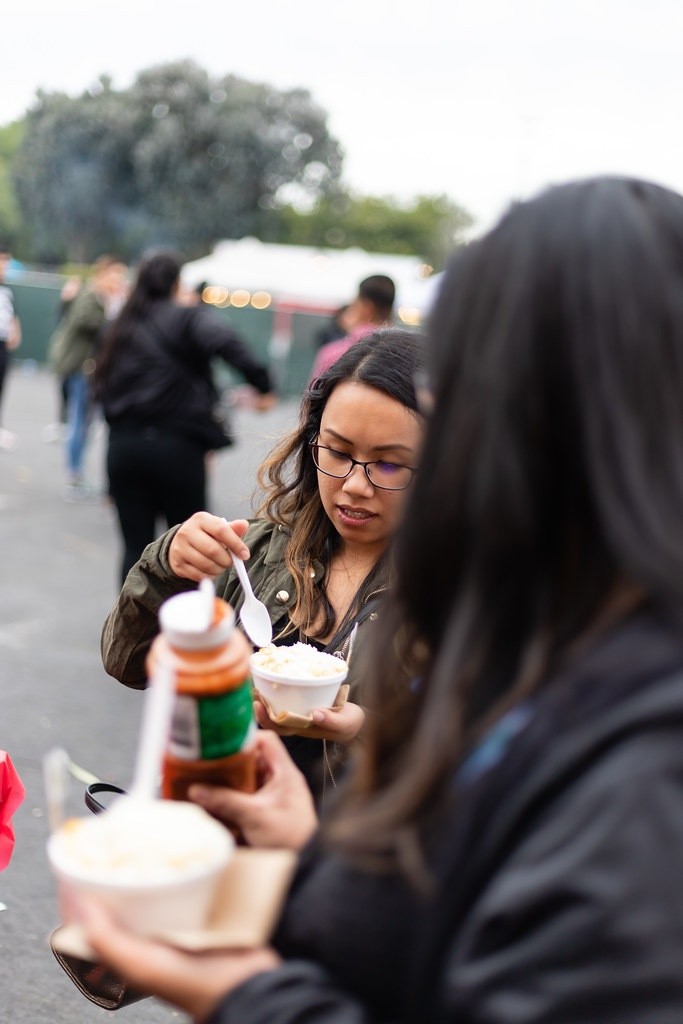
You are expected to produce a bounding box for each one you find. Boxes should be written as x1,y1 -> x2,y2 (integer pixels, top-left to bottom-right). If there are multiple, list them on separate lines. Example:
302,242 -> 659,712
146,579 -> 259,840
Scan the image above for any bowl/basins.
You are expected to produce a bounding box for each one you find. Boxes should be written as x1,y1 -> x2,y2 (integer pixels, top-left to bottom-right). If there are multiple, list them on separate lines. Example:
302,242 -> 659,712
43,821 -> 234,939
249,653 -> 348,717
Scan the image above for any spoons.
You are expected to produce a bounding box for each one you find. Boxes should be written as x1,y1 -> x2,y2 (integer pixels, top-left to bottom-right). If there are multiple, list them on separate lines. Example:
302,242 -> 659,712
221,518 -> 273,648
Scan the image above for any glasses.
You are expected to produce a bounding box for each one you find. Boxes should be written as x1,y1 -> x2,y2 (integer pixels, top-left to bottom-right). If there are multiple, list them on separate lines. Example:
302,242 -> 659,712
308,429 -> 418,491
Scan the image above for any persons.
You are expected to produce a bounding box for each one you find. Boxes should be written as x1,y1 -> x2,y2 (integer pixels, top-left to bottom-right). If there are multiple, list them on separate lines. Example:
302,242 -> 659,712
49,254 -> 436,802
57,174 -> 683,1024
0,248 -> 21,425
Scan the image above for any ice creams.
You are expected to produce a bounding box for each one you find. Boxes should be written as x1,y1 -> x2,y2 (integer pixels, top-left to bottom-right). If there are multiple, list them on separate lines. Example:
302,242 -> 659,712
44,798 -> 231,945
249,641 -> 349,718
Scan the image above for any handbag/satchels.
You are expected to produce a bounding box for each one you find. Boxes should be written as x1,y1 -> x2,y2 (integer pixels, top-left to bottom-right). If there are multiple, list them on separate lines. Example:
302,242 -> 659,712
50,782 -> 154,1012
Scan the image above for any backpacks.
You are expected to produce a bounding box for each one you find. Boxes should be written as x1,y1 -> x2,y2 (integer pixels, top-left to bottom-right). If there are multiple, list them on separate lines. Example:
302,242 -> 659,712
93,306 -> 199,430
